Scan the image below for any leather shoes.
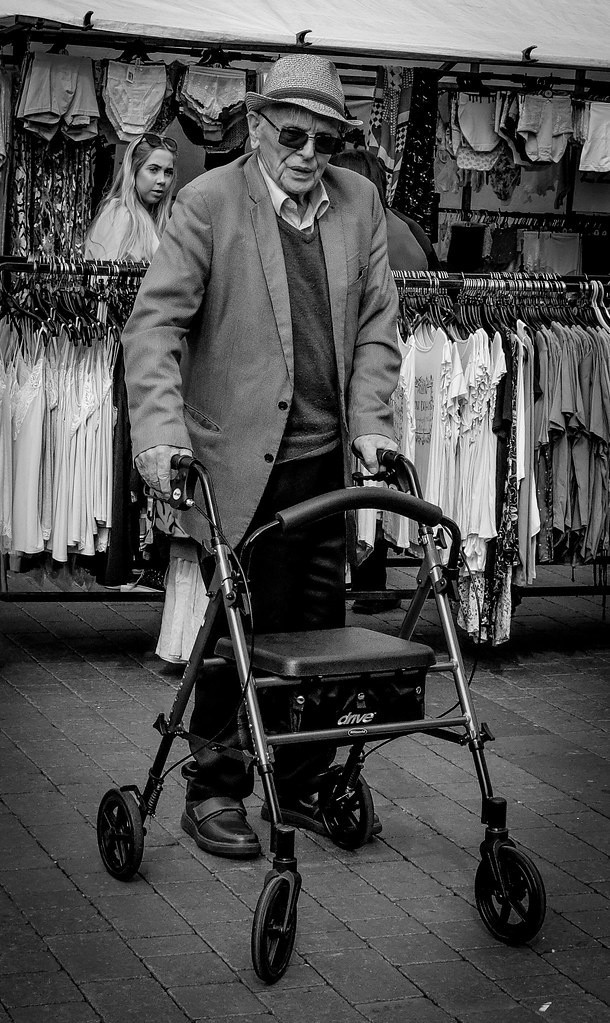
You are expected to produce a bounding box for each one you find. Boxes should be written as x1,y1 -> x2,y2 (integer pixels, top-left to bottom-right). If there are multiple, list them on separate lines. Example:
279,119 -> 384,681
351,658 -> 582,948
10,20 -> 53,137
180,795 -> 262,855
262,786 -> 382,839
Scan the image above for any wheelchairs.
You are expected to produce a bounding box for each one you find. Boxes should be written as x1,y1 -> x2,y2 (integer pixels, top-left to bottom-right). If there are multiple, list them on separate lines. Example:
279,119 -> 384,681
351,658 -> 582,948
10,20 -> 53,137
97,453 -> 546,982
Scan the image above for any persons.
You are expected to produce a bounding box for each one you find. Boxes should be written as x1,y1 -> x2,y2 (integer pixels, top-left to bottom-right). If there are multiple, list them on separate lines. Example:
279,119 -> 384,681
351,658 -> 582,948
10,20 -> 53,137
329,150 -> 429,270
119,54 -> 404,856
85,132 -> 178,263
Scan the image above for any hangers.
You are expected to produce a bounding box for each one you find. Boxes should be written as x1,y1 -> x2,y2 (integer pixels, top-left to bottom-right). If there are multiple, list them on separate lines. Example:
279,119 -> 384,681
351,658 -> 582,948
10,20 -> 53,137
0,32 -> 610,369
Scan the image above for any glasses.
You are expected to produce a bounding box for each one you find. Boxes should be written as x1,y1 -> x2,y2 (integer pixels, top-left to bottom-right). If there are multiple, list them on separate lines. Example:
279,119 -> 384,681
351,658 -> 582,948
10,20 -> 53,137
132,132 -> 178,157
259,111 -> 345,156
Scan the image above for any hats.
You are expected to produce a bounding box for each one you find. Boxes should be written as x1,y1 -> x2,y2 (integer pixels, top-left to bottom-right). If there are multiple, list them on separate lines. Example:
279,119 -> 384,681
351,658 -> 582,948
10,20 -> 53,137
246,55 -> 363,133
